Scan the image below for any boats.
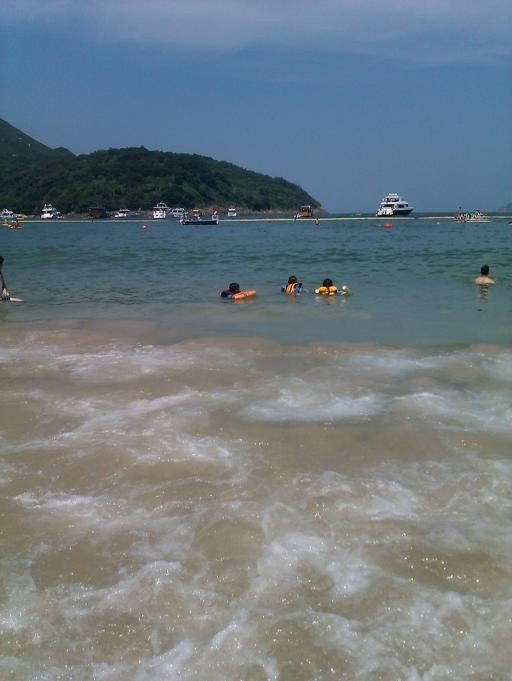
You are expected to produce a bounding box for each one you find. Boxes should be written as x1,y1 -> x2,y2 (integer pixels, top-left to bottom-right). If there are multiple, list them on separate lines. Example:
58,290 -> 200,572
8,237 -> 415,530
1,209 -> 24,229
152,202 -> 218,225
115,208 -> 130,217
455,207 -> 490,222
228,209 -> 236,217
88,208 -> 106,218
41,204 -> 62,218
296,205 -> 314,218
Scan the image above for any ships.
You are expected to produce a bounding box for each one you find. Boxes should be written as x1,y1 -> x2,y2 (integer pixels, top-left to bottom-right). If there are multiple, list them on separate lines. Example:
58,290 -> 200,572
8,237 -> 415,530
375,192 -> 413,216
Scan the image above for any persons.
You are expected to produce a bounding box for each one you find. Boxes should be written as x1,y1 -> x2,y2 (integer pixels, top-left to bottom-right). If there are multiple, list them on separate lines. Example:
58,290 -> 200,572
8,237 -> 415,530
474,265 -> 495,283
315,218 -> 320,225
220,282 -> 240,300
316,278 -> 339,295
0,255 -> 10,301
281,276 -> 303,293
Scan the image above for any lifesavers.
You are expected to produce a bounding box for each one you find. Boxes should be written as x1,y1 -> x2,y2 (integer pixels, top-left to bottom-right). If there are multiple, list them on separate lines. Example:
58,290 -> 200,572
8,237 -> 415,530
384,223 -> 392,228
234,290 -> 256,300
